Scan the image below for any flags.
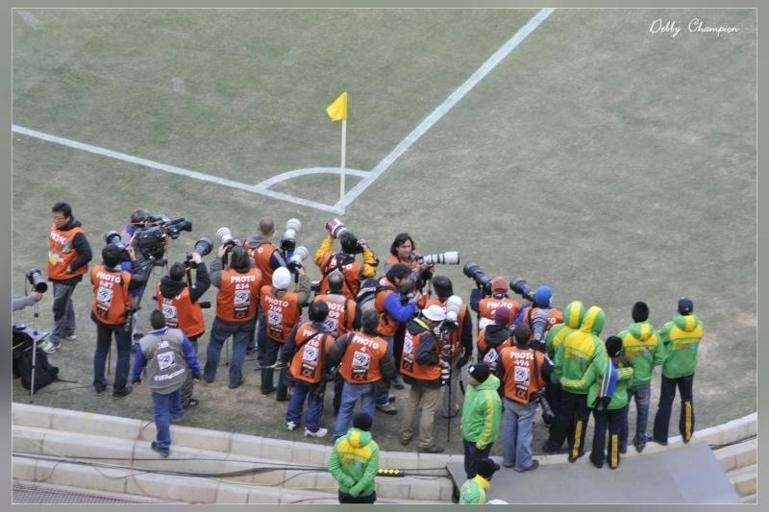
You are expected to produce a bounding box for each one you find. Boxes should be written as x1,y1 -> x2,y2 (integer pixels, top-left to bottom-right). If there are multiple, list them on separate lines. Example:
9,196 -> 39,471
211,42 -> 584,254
326,92 -> 349,122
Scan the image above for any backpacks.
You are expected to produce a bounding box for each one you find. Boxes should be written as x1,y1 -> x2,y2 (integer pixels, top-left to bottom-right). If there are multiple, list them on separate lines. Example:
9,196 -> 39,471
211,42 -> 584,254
20,348 -> 59,390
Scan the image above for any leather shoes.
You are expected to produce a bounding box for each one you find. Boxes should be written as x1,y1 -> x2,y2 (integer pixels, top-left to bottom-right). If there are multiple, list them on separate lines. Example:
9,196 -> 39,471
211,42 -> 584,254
401,439 -> 407,446
417,446 -> 444,453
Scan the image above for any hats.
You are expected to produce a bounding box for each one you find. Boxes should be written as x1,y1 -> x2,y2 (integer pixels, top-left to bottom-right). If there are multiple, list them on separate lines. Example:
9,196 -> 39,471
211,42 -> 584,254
467,364 -> 488,383
632,302 -> 649,322
606,337 -> 622,357
492,277 -> 508,292
678,297 -> 693,313
353,413 -> 371,430
422,304 -> 446,321
495,307 -> 510,325
478,458 -> 496,477
271,266 -> 291,289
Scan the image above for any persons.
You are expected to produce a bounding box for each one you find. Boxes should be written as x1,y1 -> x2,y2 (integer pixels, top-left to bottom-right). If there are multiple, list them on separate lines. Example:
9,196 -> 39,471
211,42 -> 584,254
647,297 -> 704,445
157,251 -> 211,409
618,301 -> 666,454
204,218 -> 472,452
459,458 -> 500,505
11,290 -> 43,312
90,244 -> 148,400
329,412 -> 379,505
461,363 -> 501,479
42,202 -> 93,355
470,277 -> 633,469
131,309 -> 202,457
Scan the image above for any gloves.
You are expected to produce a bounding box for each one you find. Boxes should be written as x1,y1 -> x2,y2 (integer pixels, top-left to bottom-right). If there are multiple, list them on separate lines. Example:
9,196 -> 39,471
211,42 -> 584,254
595,397 -> 610,417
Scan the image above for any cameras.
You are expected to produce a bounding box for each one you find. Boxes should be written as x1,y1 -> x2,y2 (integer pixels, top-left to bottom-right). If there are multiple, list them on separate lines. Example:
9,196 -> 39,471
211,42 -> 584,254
439,345 -> 451,387
26,268 -> 47,293
103,230 -> 132,260
510,276 -> 536,303
326,217 -> 362,253
418,251 -> 460,267
287,246 -> 309,274
444,295 -> 463,329
216,226 -> 242,252
183,237 -> 214,271
531,313 -> 548,351
279,217 -> 301,254
463,262 -> 493,297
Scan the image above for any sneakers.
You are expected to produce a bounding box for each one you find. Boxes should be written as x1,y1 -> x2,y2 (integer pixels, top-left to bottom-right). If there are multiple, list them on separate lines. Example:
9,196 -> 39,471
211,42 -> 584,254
43,342 -> 62,354
304,426 -> 328,438
152,441 -> 169,457
376,403 -> 398,415
187,399 -> 199,408
388,394 -> 396,403
286,420 -> 296,432
67,335 -> 77,341
543,443 -> 559,453
526,460 -> 538,470
113,387 -> 133,397
